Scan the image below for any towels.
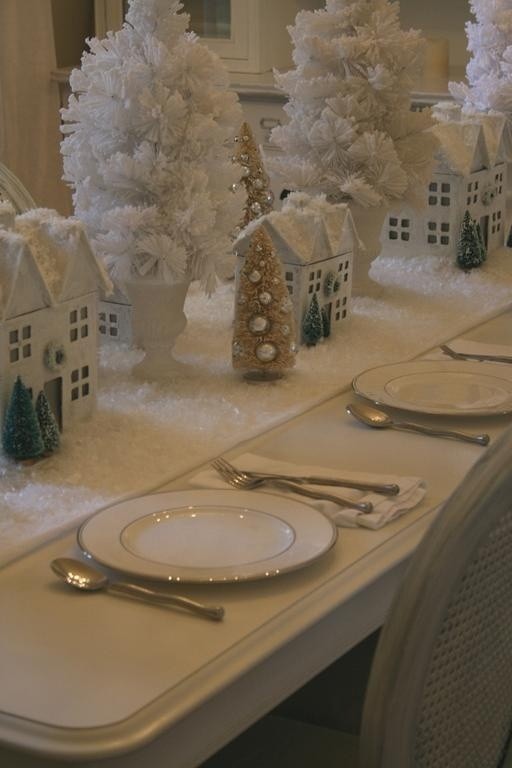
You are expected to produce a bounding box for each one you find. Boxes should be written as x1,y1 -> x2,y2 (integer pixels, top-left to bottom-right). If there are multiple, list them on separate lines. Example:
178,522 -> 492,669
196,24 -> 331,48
189,450 -> 428,530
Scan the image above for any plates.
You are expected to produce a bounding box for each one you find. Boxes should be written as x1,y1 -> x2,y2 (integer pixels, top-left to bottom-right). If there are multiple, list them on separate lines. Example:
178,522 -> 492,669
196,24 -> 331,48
77,487 -> 339,585
352,357 -> 511,418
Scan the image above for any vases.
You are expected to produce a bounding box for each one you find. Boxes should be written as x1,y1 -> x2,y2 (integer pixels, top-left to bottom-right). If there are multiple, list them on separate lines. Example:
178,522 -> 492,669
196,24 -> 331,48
351,209 -> 389,293
123,278 -> 196,376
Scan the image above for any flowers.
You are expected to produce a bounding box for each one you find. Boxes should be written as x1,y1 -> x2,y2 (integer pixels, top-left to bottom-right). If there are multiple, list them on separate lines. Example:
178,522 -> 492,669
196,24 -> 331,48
58,0 -> 248,298
266,1 -> 447,211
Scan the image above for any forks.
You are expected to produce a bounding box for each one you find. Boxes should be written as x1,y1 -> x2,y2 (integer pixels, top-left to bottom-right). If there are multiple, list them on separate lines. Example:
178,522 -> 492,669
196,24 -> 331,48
439,342 -> 511,364
210,456 -> 373,514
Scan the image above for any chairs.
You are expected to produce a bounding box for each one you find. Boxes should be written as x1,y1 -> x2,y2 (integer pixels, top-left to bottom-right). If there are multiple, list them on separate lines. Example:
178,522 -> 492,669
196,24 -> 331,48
361,426 -> 510,768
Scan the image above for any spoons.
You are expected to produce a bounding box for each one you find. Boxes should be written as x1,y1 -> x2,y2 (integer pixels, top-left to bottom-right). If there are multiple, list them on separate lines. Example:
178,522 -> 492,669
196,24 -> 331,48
347,402 -> 489,449
50,555 -> 225,625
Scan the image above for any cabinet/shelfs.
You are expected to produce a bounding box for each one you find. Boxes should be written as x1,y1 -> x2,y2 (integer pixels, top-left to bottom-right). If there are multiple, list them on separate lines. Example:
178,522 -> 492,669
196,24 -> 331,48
50,0 -> 464,206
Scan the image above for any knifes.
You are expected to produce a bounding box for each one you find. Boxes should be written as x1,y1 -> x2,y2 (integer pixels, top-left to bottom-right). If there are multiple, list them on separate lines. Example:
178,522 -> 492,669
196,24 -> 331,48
220,468 -> 401,498
